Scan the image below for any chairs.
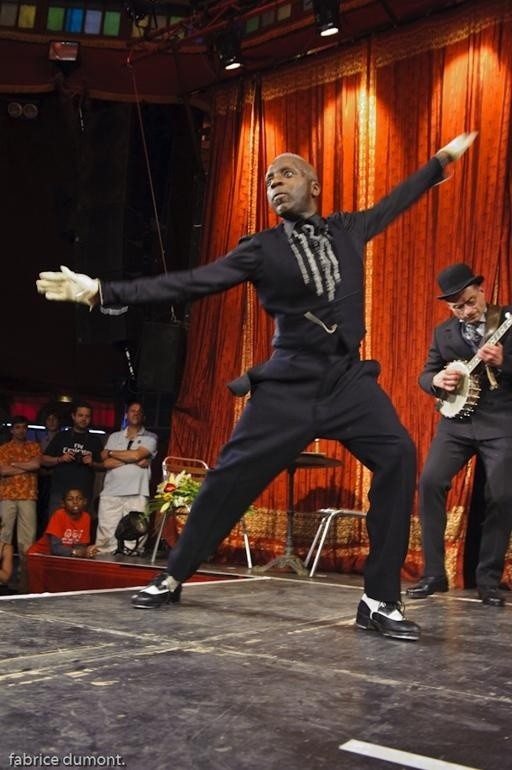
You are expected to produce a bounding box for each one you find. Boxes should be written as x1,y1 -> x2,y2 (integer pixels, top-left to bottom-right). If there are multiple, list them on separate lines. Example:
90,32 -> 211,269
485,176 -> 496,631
151,454 -> 254,570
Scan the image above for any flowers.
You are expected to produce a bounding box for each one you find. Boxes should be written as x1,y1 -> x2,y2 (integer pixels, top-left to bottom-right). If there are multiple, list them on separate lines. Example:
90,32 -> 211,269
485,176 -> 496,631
137,470 -> 255,540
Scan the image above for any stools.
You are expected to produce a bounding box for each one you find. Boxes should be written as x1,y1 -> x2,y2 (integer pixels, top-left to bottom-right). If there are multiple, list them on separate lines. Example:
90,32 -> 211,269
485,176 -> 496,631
301,507 -> 368,580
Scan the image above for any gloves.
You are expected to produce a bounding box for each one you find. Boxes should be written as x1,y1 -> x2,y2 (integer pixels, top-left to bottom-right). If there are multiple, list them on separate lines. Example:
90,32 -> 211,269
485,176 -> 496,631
438,131 -> 478,160
36,265 -> 100,312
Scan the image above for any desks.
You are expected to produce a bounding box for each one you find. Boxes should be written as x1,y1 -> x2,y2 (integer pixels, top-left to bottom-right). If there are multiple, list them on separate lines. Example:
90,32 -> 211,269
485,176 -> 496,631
248,447 -> 345,578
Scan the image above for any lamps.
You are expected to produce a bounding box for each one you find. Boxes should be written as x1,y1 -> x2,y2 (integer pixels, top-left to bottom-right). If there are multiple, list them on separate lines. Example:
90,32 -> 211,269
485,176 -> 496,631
312,1 -> 343,40
111,508 -> 148,560
212,22 -> 247,72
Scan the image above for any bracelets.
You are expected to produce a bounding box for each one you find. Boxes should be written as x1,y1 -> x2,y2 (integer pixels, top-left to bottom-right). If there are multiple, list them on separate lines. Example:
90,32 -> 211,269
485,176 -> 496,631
33,130 -> 481,642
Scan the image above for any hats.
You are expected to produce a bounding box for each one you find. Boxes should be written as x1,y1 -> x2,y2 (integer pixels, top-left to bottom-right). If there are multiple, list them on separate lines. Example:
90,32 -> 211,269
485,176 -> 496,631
436,263 -> 483,299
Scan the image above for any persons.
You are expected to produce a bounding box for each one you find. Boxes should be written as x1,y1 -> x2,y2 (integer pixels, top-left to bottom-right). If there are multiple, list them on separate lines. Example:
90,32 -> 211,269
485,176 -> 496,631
406,263 -> 512,609
0,402 -> 159,582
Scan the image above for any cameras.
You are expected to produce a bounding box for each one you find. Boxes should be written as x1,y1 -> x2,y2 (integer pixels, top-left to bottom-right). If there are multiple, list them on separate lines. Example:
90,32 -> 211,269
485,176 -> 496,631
73,451 -> 85,462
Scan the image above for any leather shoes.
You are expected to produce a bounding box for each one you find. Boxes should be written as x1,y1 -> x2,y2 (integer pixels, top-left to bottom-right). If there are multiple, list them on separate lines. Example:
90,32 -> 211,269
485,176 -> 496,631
130,571 -> 182,609
406,577 -> 448,599
356,595 -> 421,640
478,589 -> 505,607
